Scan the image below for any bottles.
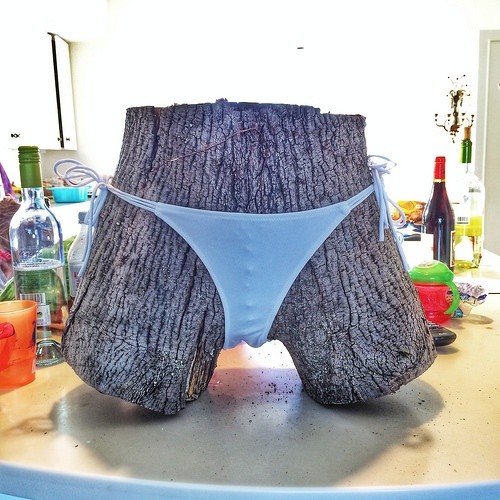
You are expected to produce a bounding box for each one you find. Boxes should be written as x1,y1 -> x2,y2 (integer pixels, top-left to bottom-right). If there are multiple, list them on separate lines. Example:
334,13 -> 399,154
421,156 -> 454,272
448,127 -> 485,268
67,212 -> 90,310
8,145 -> 68,365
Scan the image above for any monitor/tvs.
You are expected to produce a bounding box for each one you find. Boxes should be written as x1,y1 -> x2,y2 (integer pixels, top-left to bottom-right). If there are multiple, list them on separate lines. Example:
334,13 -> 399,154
475,30 -> 500,255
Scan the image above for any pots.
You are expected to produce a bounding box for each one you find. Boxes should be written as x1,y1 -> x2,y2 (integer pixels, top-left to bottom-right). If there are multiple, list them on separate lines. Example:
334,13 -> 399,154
45,187 -> 87,203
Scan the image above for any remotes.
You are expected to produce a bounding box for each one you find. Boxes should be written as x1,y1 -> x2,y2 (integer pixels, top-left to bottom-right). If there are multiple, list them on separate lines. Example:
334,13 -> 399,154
426,320 -> 457,347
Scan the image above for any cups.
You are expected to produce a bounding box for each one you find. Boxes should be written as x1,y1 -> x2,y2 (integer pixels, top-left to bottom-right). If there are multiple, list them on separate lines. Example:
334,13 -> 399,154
0,300 -> 38,389
410,259 -> 460,323
